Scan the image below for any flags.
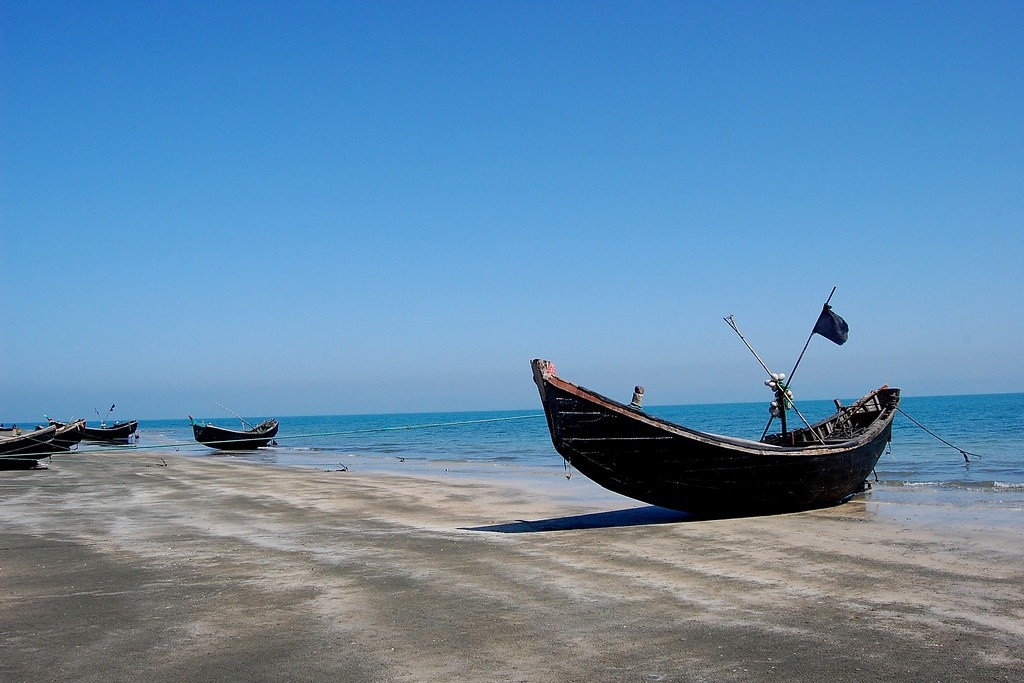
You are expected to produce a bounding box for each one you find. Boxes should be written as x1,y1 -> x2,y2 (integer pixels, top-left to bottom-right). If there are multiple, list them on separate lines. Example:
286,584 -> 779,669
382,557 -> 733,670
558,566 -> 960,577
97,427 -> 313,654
812,303 -> 850,346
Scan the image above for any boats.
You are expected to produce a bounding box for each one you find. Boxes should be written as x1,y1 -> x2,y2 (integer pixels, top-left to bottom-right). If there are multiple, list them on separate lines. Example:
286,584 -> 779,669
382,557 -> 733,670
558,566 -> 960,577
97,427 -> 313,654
528,313 -> 902,519
45,414 -> 139,442
0,416 -> 87,470
188,401 -> 280,450
